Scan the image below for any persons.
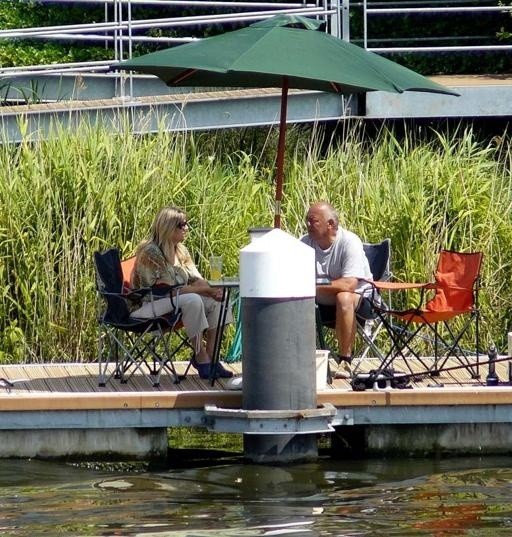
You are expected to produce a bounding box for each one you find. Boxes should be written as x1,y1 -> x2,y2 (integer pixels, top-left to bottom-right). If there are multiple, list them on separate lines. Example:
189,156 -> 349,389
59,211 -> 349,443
297,202 -> 382,378
125,207 -> 232,379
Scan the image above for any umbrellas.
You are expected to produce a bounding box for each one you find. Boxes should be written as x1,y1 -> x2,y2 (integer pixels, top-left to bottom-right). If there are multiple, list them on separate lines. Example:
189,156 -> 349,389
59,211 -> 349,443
109,13 -> 461,228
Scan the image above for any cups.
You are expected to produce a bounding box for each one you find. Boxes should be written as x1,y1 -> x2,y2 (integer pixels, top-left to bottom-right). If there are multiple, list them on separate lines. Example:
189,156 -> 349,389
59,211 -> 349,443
208,255 -> 222,281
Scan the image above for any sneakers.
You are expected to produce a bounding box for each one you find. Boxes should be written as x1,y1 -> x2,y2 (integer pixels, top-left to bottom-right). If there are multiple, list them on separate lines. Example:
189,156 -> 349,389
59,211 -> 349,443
334,360 -> 353,378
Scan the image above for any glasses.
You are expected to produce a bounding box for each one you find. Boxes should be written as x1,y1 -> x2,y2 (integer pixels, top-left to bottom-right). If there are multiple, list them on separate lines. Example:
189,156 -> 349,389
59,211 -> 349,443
177,222 -> 186,229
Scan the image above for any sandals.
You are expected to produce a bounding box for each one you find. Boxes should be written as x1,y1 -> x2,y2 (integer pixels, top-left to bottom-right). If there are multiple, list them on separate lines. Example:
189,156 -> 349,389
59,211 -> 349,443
191,353 -> 233,379
